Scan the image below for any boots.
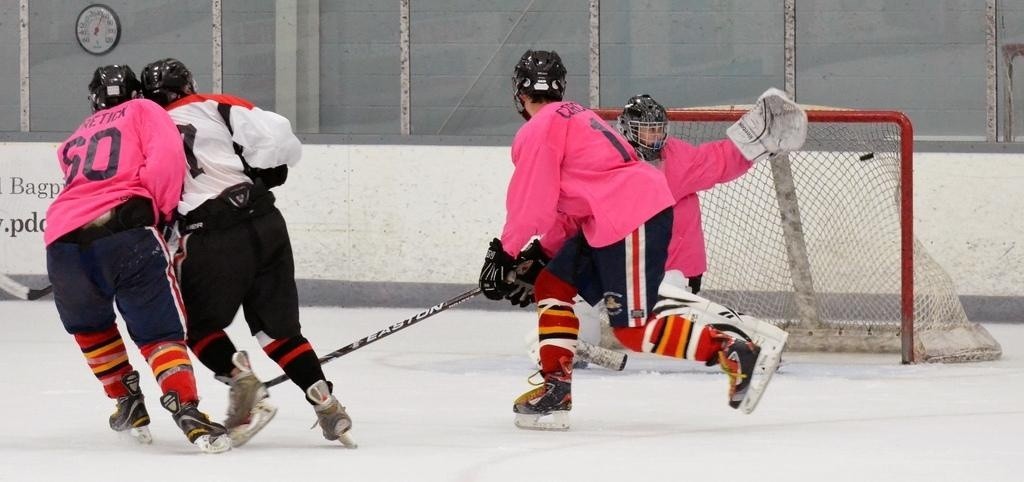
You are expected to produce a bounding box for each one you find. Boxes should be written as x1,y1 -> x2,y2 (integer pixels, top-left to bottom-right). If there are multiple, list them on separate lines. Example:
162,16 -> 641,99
705,324 -> 761,409
306,380 -> 353,441
160,390 -> 230,444
109,370 -> 151,433
513,355 -> 573,415
214,350 -> 269,433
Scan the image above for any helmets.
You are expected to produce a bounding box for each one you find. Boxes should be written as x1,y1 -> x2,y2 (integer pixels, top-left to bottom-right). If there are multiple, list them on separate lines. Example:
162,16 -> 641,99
141,58 -> 198,107
511,49 -> 568,121
88,65 -> 141,113
616,93 -> 669,163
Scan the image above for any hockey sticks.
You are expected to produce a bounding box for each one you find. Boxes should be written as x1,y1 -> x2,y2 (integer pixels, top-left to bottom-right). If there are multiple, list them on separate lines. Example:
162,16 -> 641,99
0,275 -> 53,300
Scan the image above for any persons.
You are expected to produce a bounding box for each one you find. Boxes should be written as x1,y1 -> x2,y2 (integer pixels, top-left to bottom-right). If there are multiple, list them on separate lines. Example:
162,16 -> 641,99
480,47 -> 762,415
618,85 -> 810,296
43,64 -> 225,447
140,55 -> 353,444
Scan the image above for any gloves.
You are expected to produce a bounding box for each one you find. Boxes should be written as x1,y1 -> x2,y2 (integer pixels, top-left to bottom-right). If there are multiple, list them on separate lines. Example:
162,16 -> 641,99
480,237 -> 552,307
